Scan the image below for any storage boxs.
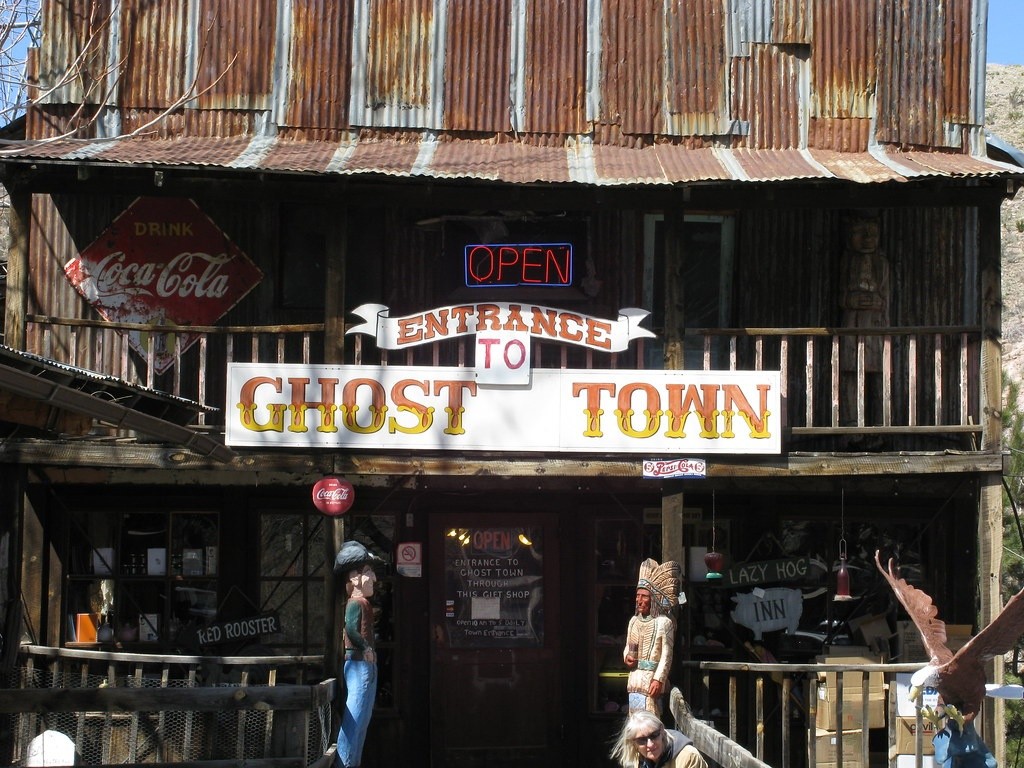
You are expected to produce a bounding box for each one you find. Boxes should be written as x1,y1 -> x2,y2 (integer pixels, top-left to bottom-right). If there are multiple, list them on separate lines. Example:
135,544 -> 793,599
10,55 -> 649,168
807,612 -> 974,768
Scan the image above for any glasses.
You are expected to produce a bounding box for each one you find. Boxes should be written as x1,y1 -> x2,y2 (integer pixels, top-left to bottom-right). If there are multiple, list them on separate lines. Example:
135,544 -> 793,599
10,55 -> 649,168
634,726 -> 662,745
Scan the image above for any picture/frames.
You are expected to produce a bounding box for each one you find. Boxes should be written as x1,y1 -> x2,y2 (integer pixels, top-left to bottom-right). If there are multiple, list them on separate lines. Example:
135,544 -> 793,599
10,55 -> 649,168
64,508 -> 220,649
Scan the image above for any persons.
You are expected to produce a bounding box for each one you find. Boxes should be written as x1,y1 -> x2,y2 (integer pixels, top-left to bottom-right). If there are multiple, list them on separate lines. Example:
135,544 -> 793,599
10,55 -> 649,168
622,557 -> 681,719
610,710 -> 709,768
828,215 -> 892,452
332,541 -> 386,768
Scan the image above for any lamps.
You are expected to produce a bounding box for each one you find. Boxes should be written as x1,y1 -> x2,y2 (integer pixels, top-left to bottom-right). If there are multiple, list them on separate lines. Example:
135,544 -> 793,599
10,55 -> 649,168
832,486 -> 854,602
704,489 -> 725,578
